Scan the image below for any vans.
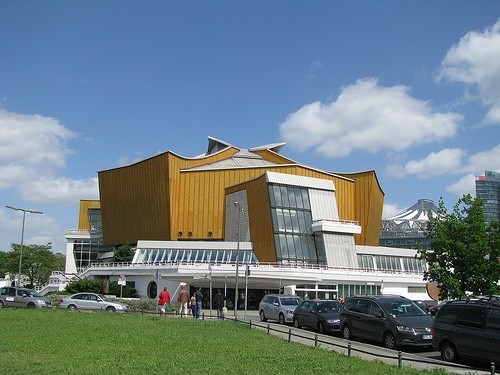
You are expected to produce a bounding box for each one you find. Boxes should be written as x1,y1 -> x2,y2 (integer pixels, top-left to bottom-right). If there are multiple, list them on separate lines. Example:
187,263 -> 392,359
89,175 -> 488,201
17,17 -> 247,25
340,295 -> 434,350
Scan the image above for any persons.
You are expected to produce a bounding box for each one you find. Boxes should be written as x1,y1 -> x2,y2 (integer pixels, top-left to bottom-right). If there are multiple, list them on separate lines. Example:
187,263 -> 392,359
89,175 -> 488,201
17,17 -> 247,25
195,286 -> 203,320
157,286 -> 171,314
191,293 -> 197,317
337,293 -> 354,303
214,289 -> 227,318
177,286 -> 191,318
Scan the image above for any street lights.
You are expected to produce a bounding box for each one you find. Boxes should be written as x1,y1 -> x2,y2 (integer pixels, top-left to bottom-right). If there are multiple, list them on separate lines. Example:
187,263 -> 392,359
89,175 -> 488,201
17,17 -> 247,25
5,205 -> 44,286
234,201 -> 241,320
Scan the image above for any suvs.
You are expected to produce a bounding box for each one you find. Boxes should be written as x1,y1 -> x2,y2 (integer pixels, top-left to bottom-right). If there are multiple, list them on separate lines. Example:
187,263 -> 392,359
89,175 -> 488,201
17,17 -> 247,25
430,299 -> 500,367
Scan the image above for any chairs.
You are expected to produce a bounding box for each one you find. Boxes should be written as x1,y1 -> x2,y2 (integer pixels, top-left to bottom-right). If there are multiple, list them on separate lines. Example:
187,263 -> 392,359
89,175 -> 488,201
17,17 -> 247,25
390,304 -> 400,314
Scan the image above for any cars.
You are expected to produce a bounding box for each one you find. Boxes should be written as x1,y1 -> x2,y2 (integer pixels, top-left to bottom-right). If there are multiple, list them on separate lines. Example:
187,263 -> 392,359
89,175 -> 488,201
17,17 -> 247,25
293,300 -> 341,335
0,286 -> 53,309
428,300 -> 451,318
58,292 -> 130,313
258,295 -> 306,324
